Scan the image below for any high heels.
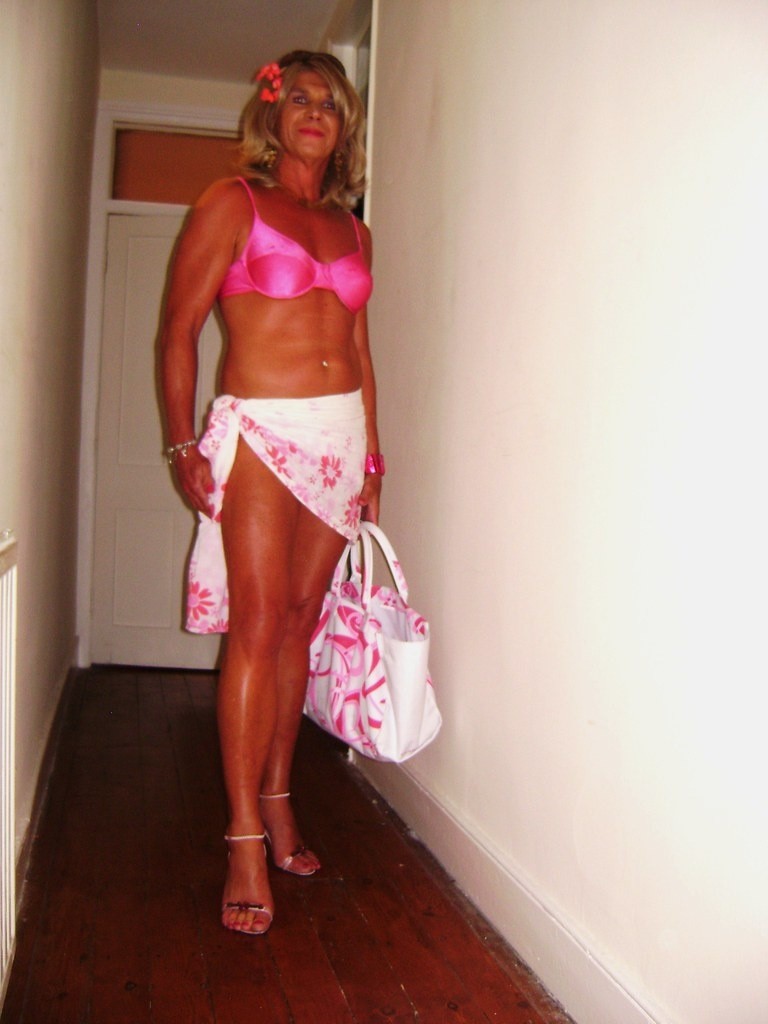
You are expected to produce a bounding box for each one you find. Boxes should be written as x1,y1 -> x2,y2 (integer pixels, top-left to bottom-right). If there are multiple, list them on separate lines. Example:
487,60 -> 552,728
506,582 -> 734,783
259,793 -> 315,876
222,830 -> 275,934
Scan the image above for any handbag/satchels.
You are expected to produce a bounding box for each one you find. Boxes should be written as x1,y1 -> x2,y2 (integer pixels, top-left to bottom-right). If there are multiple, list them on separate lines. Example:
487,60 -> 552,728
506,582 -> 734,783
302,520 -> 442,763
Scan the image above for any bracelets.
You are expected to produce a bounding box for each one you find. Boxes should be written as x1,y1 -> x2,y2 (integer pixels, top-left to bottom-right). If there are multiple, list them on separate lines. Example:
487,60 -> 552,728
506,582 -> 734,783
162,439 -> 197,466
365,453 -> 385,476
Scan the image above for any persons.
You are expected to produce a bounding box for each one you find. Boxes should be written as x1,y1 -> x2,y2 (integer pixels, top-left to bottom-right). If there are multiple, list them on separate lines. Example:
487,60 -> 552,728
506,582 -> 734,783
156,49 -> 383,935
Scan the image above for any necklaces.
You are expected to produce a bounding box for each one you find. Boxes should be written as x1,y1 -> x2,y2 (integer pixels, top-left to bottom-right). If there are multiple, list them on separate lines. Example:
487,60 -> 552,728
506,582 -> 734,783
282,183 -> 312,207
